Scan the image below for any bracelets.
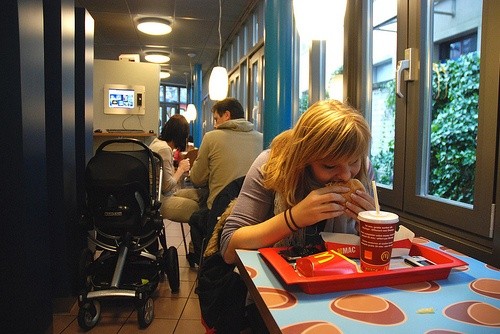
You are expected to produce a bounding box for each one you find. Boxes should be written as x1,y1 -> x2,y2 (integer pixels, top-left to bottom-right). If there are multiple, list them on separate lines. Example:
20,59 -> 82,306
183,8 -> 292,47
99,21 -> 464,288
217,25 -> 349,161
284,208 -> 298,233
289,208 -> 303,230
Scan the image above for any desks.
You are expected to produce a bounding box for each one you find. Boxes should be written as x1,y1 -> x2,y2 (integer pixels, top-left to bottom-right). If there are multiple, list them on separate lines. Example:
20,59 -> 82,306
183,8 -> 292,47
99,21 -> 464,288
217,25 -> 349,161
92,132 -> 157,137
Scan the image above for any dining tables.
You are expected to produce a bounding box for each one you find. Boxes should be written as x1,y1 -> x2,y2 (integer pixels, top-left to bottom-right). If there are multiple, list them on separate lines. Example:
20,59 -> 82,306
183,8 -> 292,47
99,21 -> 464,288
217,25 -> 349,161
231,237 -> 500,334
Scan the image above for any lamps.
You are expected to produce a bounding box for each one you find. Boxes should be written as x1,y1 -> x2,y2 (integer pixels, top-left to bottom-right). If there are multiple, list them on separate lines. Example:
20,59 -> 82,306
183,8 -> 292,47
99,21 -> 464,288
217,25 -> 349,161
292,0 -> 348,40
137,18 -> 172,36
160,70 -> 170,78
184,72 -> 190,124
208,0 -> 228,101
186,52 -> 198,121
145,52 -> 170,64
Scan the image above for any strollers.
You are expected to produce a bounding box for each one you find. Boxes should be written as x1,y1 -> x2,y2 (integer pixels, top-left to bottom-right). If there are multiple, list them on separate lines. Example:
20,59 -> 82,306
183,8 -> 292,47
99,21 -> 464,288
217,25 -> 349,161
76,138 -> 180,331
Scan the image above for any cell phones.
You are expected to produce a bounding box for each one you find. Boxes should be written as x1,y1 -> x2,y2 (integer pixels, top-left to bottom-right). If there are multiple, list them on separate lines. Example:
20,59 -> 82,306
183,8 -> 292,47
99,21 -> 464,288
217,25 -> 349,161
279,246 -> 322,260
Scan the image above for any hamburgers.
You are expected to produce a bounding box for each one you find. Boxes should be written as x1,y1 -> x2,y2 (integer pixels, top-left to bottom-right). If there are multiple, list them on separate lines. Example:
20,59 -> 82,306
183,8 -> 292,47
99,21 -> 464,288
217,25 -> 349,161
325,178 -> 366,209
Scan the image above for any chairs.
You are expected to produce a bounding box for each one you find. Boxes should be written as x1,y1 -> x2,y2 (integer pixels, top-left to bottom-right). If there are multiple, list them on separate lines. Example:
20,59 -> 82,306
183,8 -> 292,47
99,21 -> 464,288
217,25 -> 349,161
196,224 -> 270,334
187,175 -> 247,270
184,148 -> 199,170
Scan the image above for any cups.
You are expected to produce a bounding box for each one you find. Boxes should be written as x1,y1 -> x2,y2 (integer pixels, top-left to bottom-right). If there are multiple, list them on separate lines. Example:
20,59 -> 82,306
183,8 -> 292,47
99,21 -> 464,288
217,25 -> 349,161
357,211 -> 399,272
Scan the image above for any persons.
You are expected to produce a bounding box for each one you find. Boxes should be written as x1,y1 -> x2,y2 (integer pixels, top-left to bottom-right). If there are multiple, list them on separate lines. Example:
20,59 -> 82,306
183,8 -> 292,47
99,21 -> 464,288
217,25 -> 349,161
149,115 -> 203,222
221,99 -> 380,264
191,98 -> 263,265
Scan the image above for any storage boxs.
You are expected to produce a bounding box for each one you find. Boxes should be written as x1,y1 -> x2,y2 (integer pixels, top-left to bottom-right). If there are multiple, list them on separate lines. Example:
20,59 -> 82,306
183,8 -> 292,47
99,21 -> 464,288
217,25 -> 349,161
296,224 -> 415,279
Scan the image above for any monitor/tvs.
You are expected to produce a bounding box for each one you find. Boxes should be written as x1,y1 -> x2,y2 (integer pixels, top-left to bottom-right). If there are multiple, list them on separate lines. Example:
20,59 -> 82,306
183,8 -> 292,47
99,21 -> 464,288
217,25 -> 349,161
103,84 -> 145,115
